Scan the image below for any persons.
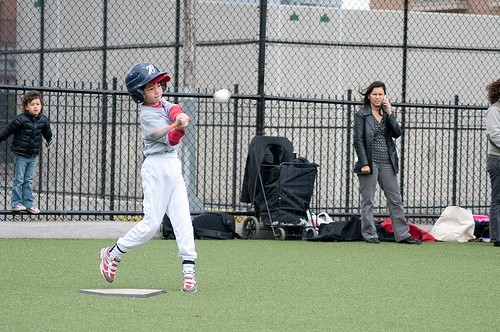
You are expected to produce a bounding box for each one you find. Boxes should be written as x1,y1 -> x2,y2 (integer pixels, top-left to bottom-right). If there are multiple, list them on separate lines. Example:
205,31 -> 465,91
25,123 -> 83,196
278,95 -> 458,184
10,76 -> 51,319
352,80 -> 422,244
484,79 -> 500,247
0,91 -> 53,214
99,62 -> 198,293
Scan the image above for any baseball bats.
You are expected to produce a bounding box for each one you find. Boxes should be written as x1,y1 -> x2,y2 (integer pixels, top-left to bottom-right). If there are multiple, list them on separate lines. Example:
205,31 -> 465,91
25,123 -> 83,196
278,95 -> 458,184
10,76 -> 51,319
145,118 -> 193,141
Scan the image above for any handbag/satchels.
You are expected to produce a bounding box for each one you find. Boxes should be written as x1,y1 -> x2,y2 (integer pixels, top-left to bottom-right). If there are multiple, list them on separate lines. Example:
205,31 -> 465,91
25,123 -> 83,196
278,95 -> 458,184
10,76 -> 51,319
430,205 -> 476,243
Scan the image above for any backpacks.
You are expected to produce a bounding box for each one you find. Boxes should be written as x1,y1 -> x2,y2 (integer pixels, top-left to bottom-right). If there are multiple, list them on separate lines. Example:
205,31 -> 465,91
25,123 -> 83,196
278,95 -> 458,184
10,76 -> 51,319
160,214 -> 176,239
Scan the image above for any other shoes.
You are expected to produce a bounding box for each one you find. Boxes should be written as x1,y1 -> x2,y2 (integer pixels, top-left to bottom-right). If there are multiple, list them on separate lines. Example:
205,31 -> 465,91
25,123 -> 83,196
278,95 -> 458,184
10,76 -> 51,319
494,242 -> 500,246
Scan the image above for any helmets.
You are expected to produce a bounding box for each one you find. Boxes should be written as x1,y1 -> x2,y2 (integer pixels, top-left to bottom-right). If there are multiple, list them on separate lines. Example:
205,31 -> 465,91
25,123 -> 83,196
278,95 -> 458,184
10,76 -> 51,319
125,63 -> 171,104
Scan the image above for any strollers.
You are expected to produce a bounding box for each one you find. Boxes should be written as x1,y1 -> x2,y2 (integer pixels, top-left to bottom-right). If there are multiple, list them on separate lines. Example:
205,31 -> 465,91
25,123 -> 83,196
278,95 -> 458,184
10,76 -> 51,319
240,134 -> 319,241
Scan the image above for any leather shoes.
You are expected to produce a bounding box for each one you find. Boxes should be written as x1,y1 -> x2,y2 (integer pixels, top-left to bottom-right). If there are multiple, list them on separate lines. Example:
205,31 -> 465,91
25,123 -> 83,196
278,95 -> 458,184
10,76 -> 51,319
398,236 -> 418,244
367,238 -> 380,243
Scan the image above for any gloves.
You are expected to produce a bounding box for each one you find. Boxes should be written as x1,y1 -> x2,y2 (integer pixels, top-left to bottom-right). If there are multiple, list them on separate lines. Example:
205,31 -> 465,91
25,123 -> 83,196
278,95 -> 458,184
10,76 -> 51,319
175,113 -> 189,130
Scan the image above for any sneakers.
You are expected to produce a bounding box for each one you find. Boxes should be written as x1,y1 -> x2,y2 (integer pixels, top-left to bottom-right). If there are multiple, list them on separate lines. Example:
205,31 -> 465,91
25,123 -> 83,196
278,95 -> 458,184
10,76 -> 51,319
181,269 -> 197,294
99,246 -> 120,283
27,205 -> 40,214
10,202 -> 26,211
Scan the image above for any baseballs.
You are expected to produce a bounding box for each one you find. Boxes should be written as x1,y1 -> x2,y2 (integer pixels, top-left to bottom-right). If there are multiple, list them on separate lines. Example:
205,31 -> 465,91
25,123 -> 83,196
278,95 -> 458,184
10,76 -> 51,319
213,89 -> 232,104
